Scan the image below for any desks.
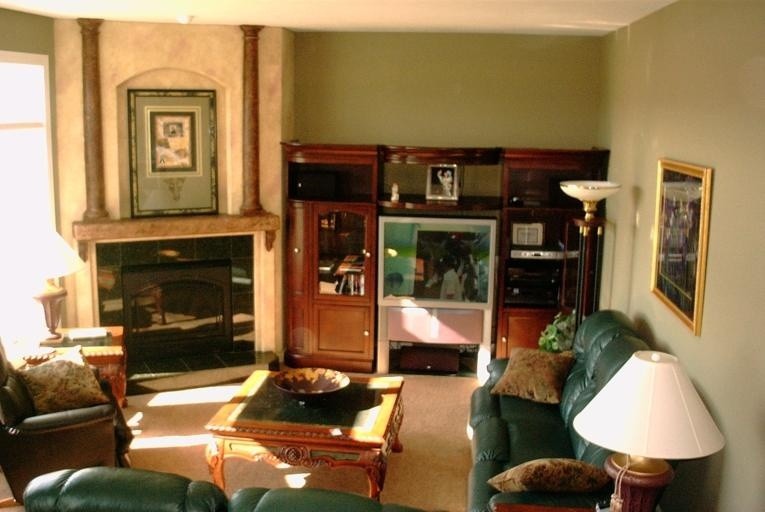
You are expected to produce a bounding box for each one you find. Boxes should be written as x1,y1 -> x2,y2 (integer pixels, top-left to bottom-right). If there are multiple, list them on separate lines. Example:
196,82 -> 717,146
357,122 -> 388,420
39,326 -> 129,406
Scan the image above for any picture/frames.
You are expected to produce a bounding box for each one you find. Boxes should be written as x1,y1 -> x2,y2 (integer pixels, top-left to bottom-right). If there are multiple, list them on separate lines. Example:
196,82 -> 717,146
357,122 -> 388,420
426,164 -> 459,201
128,86 -> 219,219
651,154 -> 715,337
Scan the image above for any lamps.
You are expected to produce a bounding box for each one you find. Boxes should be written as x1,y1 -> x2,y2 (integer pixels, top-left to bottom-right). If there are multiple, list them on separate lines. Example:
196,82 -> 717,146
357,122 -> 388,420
25,230 -> 84,339
572,351 -> 726,512
556,179 -> 622,319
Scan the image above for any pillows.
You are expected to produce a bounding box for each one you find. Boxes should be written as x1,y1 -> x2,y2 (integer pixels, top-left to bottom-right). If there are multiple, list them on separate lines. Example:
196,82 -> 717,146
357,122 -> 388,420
486,456 -> 610,493
490,346 -> 577,403
21,345 -> 109,414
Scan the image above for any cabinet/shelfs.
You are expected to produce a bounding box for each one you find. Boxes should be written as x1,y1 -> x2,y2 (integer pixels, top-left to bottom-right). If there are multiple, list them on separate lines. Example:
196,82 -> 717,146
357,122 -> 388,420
382,146 -> 503,215
495,147 -> 610,359
283,146 -> 380,372
377,307 -> 493,375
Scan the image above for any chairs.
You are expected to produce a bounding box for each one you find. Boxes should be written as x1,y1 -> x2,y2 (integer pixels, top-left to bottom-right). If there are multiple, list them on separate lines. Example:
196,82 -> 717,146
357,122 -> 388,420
0,339 -> 134,504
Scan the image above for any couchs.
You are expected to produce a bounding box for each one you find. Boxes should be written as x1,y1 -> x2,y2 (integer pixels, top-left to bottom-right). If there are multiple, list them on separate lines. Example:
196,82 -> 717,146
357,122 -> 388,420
22,465 -> 428,512
467,308 -> 654,512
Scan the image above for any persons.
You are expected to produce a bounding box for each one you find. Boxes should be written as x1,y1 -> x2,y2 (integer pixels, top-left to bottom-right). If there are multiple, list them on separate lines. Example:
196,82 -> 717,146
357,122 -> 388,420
439,256 -> 462,301
438,170 -> 453,197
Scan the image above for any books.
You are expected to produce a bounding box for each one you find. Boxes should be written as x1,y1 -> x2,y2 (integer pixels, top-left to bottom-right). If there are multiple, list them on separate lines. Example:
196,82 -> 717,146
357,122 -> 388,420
69,328 -> 107,340
319,255 -> 365,296
64,331 -> 112,346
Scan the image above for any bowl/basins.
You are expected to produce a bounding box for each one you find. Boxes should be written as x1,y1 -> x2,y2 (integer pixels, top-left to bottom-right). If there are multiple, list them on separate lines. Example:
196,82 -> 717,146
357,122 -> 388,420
268,365 -> 353,406
